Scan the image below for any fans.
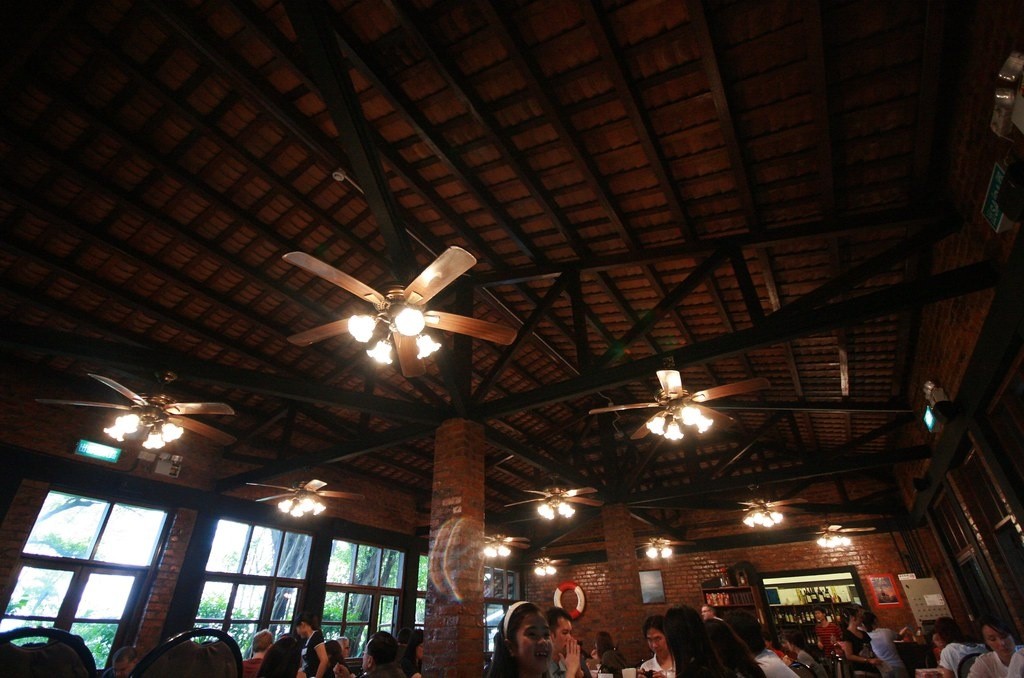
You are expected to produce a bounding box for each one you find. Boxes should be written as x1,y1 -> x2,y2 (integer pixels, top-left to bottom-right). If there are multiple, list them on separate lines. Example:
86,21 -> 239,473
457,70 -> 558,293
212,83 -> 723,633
282,245 -> 518,378
33,367 -> 237,448
801,513 -> 877,536
479,526 -> 531,549
630,526 -> 696,551
735,483 -> 807,519
244,465 -> 364,503
588,355 -> 771,440
516,547 -> 571,570
501,471 -> 605,508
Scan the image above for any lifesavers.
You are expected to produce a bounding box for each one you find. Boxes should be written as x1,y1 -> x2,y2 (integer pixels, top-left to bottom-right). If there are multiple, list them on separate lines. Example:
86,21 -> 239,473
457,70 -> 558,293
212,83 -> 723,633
553,581 -> 586,619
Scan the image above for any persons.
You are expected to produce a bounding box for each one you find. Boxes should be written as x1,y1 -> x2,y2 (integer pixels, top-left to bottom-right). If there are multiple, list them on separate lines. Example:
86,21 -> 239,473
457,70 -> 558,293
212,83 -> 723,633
813,607 -> 843,678
488,601 -> 553,678
725,608 -> 800,678
103,646 -> 137,678
664,605 -> 737,678
763,632 -> 784,659
637,615 -> 676,678
932,616 -> 985,678
578,631 -> 627,678
702,604 -> 723,621
396,627 -> 424,678
785,633 -> 828,678
546,606 -> 584,678
705,618 -> 767,678
839,604 -> 912,678
967,617 -> 1024,678
242,613 -> 406,678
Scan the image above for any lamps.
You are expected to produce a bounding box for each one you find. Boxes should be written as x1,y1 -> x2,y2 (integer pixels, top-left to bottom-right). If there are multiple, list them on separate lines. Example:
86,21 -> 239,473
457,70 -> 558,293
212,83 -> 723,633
346,306 -> 442,365
101,406 -> 184,452
740,510 -> 784,528
537,498 -> 576,520
816,533 -> 850,547
533,564 -> 558,576
646,401 -> 713,441
482,540 -> 512,558
277,490 -> 326,517
646,543 -> 672,559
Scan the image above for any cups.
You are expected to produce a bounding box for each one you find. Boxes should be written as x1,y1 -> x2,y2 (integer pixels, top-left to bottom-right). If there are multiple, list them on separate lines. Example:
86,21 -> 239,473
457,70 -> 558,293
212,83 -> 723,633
660,670 -> 675,678
622,668 -> 637,678
598,673 -> 613,678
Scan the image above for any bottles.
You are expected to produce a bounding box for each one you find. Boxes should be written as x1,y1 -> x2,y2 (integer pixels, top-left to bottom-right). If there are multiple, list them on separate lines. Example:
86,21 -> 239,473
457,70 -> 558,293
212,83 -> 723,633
706,592 -> 730,606
799,585 -> 841,604
773,607 -> 854,644
917,627 -> 926,646
718,568 -> 731,587
737,569 -> 748,587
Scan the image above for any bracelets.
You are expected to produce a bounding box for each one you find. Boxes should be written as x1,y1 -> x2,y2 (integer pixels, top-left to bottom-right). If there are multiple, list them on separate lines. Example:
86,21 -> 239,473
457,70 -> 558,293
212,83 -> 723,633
864,658 -> 870,663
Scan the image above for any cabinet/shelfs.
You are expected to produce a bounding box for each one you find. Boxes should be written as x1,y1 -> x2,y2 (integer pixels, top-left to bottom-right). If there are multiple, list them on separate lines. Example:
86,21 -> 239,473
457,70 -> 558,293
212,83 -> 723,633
698,577 -> 770,640
767,600 -> 858,653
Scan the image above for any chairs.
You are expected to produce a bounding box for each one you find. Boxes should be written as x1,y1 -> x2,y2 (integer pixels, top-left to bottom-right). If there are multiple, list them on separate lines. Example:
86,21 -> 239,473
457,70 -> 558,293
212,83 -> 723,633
126,628 -> 244,678
829,650 -> 846,678
0,625 -> 99,678
956,652 -> 982,678
